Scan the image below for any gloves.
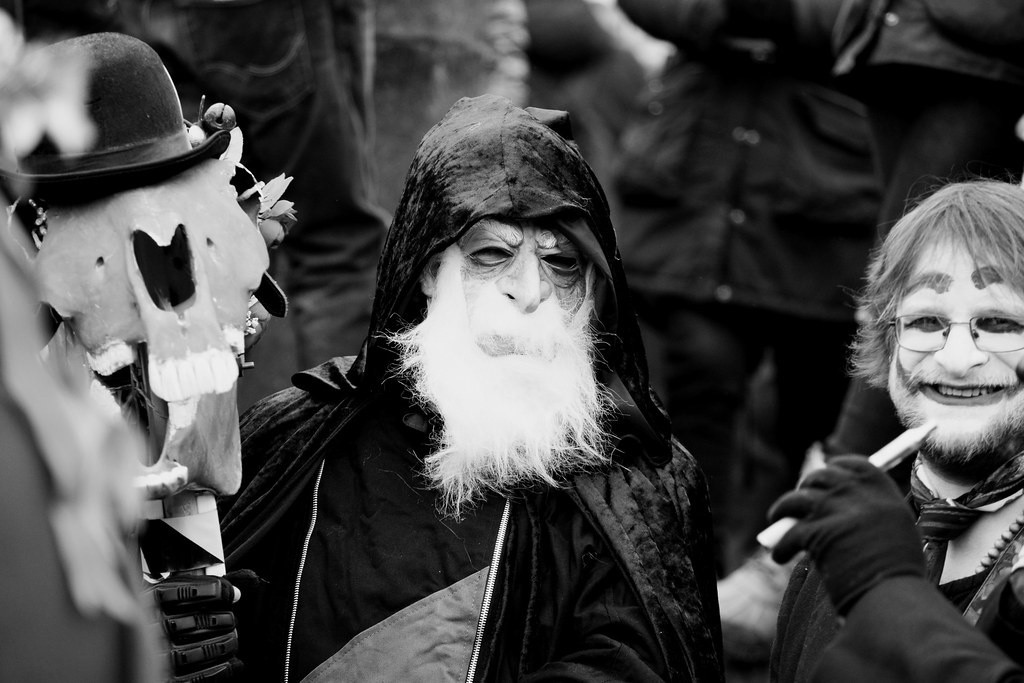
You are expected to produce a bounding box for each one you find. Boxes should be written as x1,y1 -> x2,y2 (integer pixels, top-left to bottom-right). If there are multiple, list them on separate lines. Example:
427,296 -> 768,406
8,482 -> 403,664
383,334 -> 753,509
769,454 -> 927,615
155,569 -> 288,683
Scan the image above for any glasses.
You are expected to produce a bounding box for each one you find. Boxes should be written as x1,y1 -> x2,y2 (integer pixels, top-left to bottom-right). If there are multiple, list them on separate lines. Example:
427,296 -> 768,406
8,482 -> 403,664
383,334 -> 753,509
882,313 -> 1024,353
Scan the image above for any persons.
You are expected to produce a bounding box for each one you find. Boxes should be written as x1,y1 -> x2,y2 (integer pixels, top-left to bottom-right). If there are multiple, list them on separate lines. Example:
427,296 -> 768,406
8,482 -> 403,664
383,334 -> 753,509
146,95 -> 724,683
769,178 -> 1024,683
0,0 -> 1024,572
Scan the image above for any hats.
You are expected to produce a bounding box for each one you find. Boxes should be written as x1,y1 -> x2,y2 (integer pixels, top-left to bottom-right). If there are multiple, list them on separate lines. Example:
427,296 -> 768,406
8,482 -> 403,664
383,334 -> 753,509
1,31 -> 236,209
